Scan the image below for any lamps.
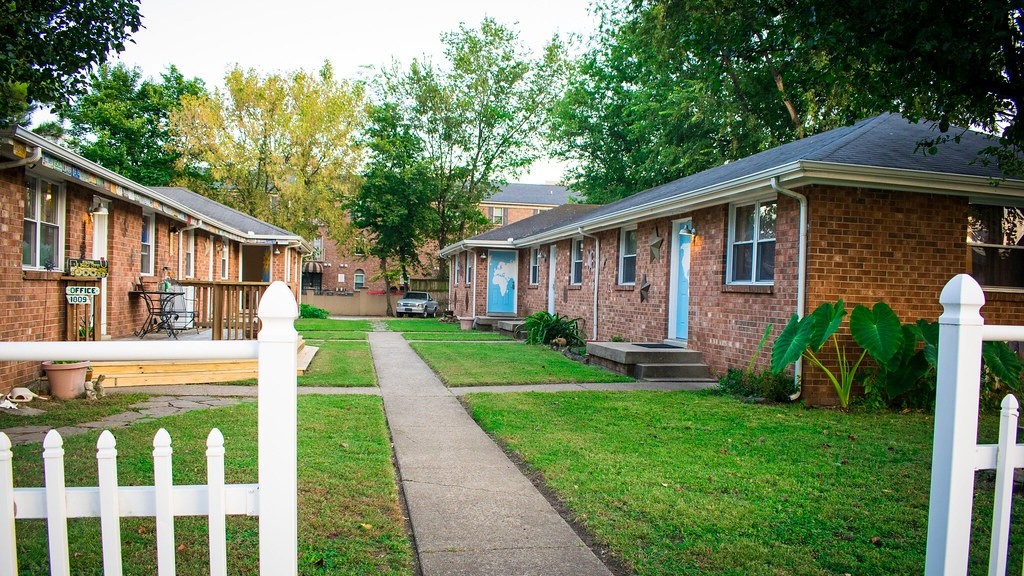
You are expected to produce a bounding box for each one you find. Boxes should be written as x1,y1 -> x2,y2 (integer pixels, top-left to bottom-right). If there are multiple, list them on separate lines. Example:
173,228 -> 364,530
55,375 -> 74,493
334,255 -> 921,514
273,247 -> 280,254
88,195 -> 110,216
537,253 -> 546,262
679,224 -> 696,236
480,252 -> 487,259
169,226 -> 181,234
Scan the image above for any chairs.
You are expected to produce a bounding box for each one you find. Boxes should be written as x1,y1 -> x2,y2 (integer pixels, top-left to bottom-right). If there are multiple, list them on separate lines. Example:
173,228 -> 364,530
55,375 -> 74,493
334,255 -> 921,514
143,276 -> 199,338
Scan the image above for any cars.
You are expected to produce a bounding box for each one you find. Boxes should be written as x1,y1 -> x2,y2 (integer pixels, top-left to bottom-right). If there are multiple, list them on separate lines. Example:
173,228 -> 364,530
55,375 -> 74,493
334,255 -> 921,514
396,291 -> 439,319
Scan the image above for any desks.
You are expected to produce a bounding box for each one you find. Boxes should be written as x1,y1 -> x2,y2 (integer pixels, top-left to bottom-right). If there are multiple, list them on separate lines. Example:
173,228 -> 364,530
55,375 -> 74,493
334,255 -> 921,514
128,291 -> 186,340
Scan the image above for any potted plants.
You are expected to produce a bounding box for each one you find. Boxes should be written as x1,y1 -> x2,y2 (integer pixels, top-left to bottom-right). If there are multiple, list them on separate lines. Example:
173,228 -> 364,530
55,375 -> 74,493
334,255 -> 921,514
42,360 -> 90,401
79,317 -> 94,341
